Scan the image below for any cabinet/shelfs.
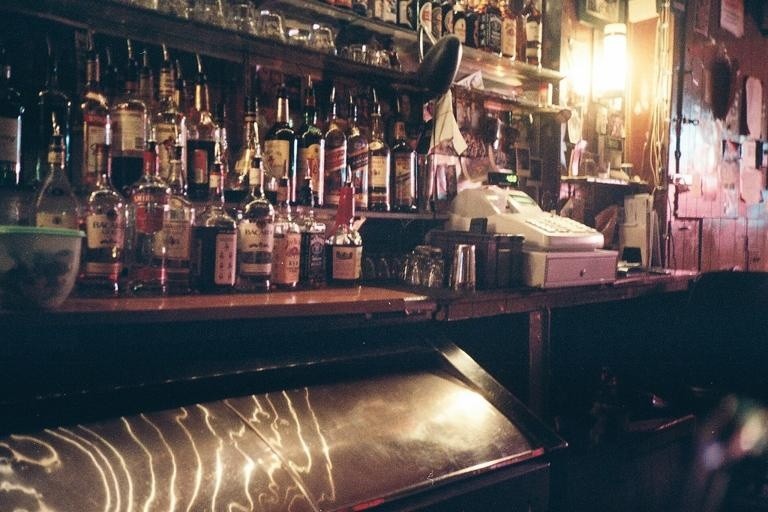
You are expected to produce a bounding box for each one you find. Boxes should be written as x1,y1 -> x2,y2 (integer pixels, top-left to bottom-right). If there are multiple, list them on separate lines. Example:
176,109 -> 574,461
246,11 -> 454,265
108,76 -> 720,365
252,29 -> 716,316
0,0 -> 565,231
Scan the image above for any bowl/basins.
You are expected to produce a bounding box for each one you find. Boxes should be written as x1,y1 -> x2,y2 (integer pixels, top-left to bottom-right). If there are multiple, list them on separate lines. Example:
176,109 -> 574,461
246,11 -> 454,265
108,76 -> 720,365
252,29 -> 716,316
0,225 -> 86,307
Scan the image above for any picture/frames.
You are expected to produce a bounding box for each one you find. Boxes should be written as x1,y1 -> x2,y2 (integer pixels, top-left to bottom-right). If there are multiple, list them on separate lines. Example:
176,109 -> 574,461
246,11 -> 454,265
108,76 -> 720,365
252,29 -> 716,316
569,0 -> 629,26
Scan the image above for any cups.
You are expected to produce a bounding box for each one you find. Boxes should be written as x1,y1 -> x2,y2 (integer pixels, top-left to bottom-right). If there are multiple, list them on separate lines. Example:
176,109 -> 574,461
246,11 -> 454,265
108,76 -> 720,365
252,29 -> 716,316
400,246 -> 444,288
446,240 -> 479,293
130,1 -> 382,63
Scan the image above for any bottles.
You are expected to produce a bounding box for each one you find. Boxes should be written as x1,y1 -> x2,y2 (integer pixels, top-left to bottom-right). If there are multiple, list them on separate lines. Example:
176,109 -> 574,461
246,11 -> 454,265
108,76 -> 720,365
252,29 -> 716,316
237,154 -> 272,292
271,172 -> 300,293
32,133 -> 81,228
292,177 -> 327,292
195,160 -> 237,295
327,180 -> 363,290
0,27 -> 425,215
166,143 -> 195,299
129,140 -> 168,296
81,142 -> 125,297
325,1 -> 542,66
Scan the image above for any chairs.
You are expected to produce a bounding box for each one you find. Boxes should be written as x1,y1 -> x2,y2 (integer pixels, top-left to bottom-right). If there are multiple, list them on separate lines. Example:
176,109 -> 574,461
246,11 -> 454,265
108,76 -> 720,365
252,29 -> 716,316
626,270 -> 767,512
